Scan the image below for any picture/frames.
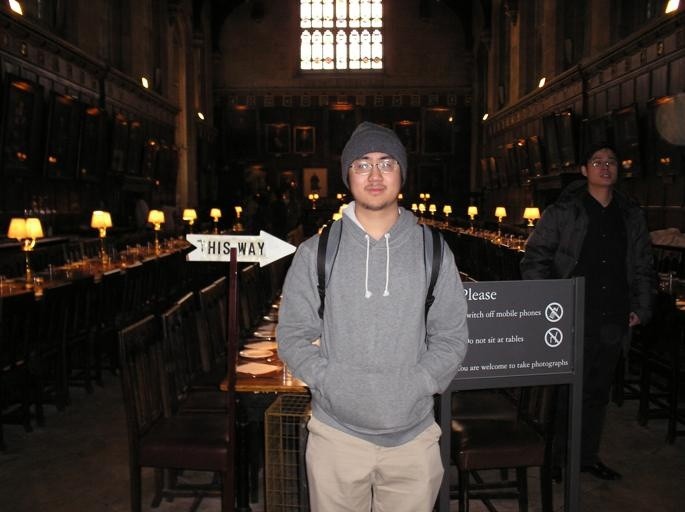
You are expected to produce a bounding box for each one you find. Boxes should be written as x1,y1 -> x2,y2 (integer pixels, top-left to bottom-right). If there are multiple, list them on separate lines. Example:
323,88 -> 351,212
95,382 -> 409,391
479,91 -> 685,190
1,71 -> 181,192
223,107 -> 455,201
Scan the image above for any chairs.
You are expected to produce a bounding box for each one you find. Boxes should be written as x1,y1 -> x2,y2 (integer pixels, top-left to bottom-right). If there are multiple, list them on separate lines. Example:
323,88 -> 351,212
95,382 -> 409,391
0,222 -> 306,511
428,225 -> 684,512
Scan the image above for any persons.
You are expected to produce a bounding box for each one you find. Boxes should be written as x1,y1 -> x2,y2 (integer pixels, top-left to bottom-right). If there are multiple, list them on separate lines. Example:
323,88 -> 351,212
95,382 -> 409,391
275,119 -> 474,511
311,172 -> 321,193
129,192 -> 151,230
248,185 -> 313,224
518,140 -> 657,485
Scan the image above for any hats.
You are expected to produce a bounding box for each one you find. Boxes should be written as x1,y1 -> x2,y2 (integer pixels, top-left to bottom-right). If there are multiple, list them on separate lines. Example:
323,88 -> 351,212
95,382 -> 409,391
341,121 -> 408,192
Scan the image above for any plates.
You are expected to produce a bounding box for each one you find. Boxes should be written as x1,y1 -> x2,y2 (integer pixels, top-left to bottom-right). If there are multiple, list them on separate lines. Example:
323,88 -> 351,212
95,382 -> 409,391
238,300 -> 280,375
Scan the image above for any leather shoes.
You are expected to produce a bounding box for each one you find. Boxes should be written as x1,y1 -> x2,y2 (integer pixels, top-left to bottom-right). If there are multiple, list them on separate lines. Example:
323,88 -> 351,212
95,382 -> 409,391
551,466 -> 562,485
580,462 -> 623,480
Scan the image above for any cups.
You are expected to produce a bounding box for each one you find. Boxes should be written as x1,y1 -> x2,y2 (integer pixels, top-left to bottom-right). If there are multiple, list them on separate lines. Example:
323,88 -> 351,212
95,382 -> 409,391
419,217 -> 525,255
1,236 -> 175,294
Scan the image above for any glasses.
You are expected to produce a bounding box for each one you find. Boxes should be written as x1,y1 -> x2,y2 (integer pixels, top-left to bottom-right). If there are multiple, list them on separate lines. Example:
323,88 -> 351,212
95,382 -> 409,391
590,159 -> 617,169
349,159 -> 399,175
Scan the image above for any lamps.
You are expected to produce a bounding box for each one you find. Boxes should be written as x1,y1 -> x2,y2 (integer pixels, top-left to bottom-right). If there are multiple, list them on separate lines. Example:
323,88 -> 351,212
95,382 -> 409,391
407,191 -> 545,235
5,204 -> 243,284
